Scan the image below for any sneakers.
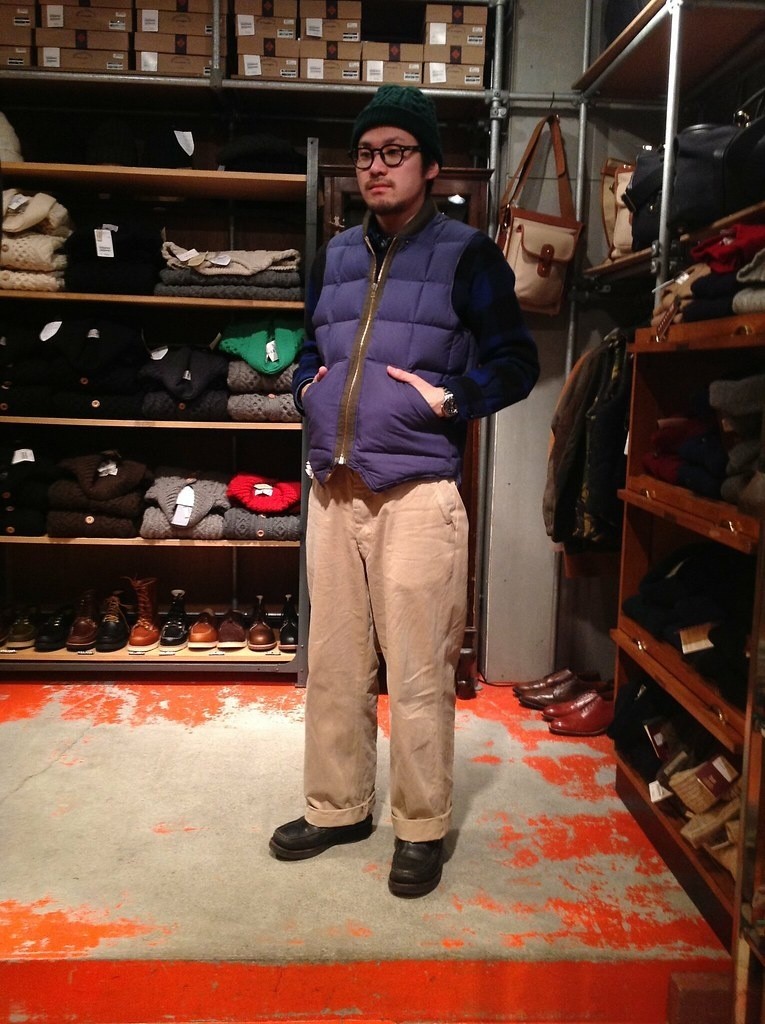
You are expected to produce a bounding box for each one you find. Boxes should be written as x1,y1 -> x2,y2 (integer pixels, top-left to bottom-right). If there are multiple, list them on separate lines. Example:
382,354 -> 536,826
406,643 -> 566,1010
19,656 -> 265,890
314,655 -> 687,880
218,610 -> 248,648
35,607 -> 72,648
187,609 -> 217,648
6,613 -> 37,647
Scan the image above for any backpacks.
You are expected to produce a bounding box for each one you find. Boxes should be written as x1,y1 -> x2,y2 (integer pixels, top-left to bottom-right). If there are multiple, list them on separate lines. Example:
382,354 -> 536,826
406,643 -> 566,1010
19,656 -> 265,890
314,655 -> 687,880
621,126 -> 742,251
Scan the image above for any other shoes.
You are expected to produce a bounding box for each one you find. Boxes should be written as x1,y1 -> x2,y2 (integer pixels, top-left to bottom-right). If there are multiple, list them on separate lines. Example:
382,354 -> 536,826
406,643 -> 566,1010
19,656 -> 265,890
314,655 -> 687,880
0,622 -> 8,642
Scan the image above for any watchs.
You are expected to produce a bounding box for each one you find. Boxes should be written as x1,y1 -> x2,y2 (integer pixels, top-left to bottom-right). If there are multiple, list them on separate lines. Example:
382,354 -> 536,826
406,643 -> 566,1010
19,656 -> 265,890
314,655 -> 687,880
441,385 -> 458,417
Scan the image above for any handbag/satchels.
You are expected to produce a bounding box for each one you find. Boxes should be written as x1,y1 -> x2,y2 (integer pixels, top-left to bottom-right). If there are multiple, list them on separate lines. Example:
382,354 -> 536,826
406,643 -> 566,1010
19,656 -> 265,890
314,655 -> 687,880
494,113 -> 584,318
602,156 -> 634,248
609,163 -> 639,250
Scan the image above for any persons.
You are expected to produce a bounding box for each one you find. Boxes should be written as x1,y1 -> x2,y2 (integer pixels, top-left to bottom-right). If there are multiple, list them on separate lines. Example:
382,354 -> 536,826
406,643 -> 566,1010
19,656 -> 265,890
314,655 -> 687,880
268,80 -> 541,898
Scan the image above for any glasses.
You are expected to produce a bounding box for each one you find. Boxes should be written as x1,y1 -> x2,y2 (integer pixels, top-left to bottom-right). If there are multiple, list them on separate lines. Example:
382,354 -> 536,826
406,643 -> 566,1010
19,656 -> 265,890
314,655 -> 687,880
354,145 -> 424,167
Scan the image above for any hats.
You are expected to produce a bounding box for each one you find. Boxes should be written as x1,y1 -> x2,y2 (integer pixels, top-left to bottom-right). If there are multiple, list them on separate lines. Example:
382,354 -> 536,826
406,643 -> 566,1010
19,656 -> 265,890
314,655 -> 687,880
352,83 -> 445,172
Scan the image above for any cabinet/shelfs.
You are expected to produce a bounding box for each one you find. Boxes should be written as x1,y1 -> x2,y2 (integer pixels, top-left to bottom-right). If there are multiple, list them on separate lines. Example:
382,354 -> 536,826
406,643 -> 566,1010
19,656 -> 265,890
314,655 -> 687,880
0,136 -> 323,684
318,162 -> 497,697
572,4 -> 764,976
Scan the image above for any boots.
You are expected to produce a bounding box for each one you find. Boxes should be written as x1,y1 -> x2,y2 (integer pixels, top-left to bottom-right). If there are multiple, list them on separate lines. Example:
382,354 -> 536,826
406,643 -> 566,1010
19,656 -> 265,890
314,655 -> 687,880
123,574 -> 161,651
279,593 -> 298,650
248,593 -> 277,650
161,589 -> 193,645
66,591 -> 100,649
96,591 -> 130,650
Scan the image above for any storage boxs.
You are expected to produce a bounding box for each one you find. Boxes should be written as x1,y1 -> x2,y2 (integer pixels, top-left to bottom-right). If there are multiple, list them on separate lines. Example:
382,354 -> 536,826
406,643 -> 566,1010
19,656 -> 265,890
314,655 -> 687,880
0,0 -> 490,89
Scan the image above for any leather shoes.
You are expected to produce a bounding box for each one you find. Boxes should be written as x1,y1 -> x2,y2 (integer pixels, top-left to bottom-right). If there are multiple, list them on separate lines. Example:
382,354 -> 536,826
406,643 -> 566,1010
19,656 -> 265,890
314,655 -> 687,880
388,837 -> 443,894
519,674 -> 610,709
513,666 -> 601,694
549,695 -> 615,735
269,814 -> 373,859
541,689 -> 613,719
